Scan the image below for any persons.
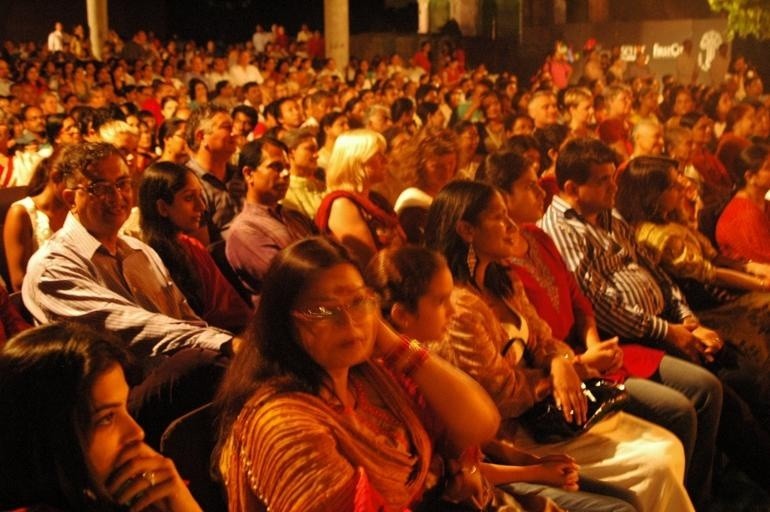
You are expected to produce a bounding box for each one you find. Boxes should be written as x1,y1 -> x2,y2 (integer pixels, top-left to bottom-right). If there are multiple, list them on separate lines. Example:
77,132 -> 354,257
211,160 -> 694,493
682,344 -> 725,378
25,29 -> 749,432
2,145 -> 86,290
475,151 -> 722,491
1,323 -> 204,512
715,144 -> 770,264
0,23 -> 257,159
424,179 -> 697,512
615,155 -> 767,291
663,126 -> 697,177
21,143 -> 243,419
456,121 -> 485,183
564,40 -> 769,132
208,237 -> 561,512
535,137 -> 770,462
225,135 -> 316,314
314,129 -> 406,271
258,26 -> 562,134
382,126 -> 412,152
535,124 -> 572,180
413,131 -> 457,196
139,162 -> 254,331
369,240 -> 643,510
500,134 -> 539,177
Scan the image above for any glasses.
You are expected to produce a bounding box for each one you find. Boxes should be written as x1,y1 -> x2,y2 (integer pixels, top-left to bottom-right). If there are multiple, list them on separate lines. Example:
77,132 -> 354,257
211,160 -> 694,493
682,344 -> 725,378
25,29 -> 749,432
286,289 -> 375,319
72,178 -> 131,197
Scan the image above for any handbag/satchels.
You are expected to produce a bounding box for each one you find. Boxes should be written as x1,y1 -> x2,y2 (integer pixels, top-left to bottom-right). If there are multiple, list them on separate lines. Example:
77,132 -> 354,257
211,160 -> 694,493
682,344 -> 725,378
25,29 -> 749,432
498,336 -> 630,445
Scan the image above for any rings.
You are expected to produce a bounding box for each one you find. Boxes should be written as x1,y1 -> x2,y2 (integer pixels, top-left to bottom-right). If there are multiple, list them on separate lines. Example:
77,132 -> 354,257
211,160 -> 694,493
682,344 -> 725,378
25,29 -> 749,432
142,469 -> 156,485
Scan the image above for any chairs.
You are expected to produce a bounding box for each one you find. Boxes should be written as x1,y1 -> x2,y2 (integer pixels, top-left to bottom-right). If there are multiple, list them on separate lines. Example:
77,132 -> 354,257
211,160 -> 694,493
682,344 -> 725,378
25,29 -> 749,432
202,239 -> 256,319
159,391 -> 233,511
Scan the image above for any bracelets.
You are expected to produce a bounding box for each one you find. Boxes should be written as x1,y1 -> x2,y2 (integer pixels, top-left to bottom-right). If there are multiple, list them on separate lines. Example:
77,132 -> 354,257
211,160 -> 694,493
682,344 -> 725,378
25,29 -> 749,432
383,333 -> 431,378
